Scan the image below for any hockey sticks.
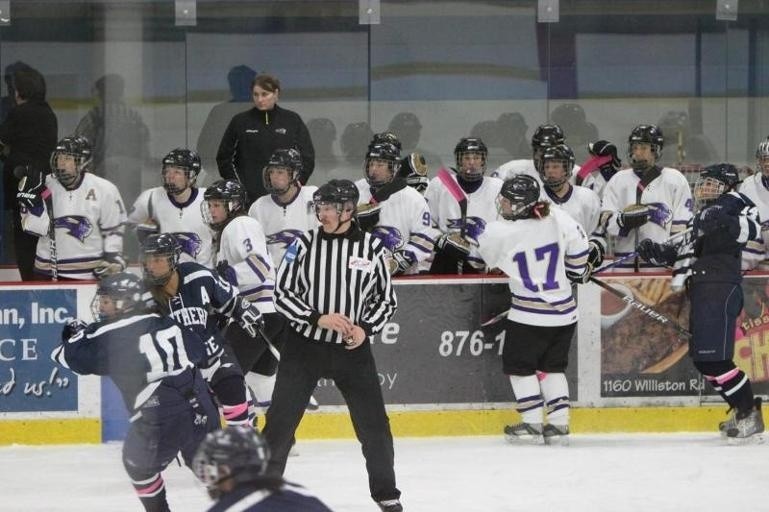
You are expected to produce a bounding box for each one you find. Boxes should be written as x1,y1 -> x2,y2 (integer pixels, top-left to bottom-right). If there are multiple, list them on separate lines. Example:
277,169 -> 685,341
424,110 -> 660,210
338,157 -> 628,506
12,166 -> 59,283
575,155 -> 610,185
481,228 -> 692,328
635,166 -> 661,271
436,168 -> 468,275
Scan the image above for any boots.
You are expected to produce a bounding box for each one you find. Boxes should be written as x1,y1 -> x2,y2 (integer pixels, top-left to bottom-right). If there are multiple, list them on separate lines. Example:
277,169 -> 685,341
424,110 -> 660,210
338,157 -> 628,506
719,408 -> 740,432
727,397 -> 764,438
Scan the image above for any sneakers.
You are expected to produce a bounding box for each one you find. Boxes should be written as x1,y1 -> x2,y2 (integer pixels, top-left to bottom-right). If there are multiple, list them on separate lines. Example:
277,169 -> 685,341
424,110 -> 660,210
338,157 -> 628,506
543,424 -> 569,436
504,423 -> 544,435
377,500 -> 403,512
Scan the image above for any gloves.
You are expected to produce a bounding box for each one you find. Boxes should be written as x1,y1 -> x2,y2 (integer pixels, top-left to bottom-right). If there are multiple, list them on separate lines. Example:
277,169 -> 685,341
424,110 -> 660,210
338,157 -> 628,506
616,205 -> 649,231
638,238 -> 669,267
16,170 -> 45,212
434,232 -> 469,263
93,253 -> 129,280
388,251 -> 417,277
61,317 -> 87,343
587,237 -> 608,268
229,296 -> 265,341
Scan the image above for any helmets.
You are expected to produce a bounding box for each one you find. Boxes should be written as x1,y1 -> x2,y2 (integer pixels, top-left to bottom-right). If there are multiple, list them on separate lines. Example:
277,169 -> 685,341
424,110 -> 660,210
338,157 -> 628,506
90,272 -> 143,322
192,424 -> 271,499
262,147 -> 303,197
200,178 -> 245,232
363,123 -> 574,220
627,124 -> 663,168
49,135 -> 92,180
160,147 -> 199,195
139,232 -> 181,287
756,136 -> 769,177
312,178 -> 359,215
693,163 -> 738,207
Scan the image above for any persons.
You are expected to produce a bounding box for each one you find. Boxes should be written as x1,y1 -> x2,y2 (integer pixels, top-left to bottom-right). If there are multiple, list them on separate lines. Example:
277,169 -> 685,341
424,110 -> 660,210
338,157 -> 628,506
477,173 -> 602,436
60,271 -> 221,511
135,233 -> 265,427
638,165 -> 764,438
260,178 -> 403,512
194,427 -> 333,512
